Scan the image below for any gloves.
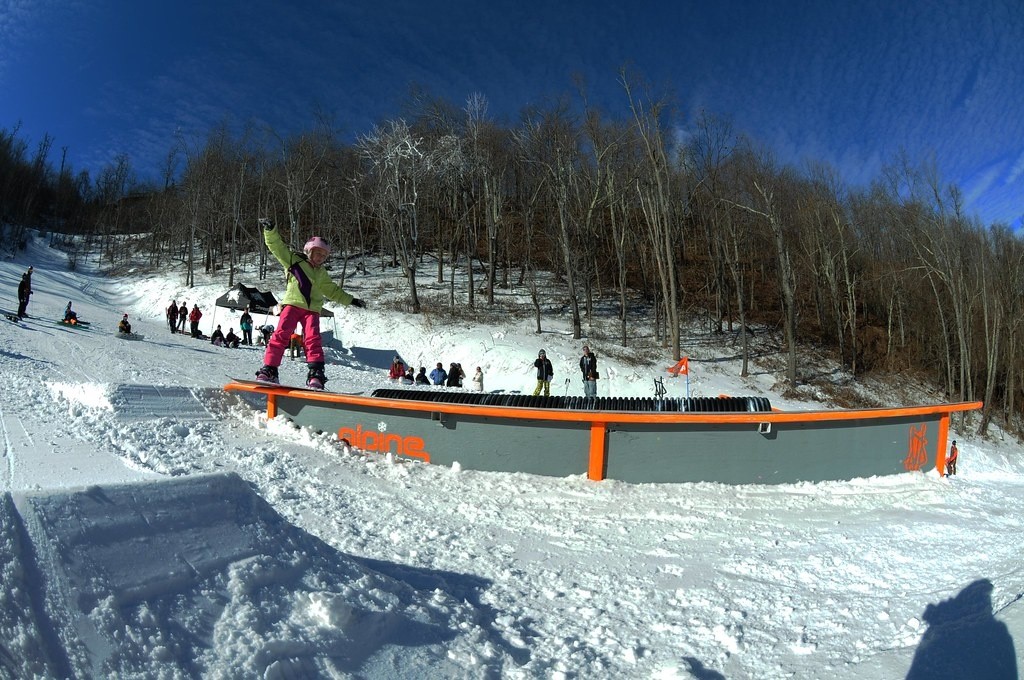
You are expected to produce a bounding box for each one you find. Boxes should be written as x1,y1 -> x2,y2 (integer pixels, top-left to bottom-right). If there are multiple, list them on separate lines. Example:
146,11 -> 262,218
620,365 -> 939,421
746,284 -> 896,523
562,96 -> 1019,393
260,218 -> 275,231
351,298 -> 366,308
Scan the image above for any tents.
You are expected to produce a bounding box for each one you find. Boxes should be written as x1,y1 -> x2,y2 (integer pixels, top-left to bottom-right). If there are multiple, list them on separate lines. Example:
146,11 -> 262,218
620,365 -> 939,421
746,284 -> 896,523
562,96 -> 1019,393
319,308 -> 337,338
212,282 -> 282,345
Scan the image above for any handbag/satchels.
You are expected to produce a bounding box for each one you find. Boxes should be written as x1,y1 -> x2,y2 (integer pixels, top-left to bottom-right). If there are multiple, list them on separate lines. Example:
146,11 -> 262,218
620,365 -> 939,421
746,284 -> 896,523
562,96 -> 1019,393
588,372 -> 600,379
548,375 -> 553,381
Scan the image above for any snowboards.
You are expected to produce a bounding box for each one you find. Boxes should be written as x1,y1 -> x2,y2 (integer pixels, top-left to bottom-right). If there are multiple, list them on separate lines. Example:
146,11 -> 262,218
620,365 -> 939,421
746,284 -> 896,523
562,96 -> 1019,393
223,373 -> 365,396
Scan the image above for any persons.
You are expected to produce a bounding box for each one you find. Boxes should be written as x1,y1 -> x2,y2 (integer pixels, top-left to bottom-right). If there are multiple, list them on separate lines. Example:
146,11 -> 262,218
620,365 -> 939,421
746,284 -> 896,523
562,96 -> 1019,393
255,217 -> 366,388
429,362 -> 466,388
167,300 -> 188,334
17,265 -> 33,321
945,440 -> 958,475
227,328 -> 239,347
64,301 -> 78,325
189,304 -> 202,337
390,355 -> 431,385
291,334 -> 303,360
579,346 -> 597,397
472,366 -> 483,392
240,308 -> 253,346
533,349 -> 553,395
119,314 -> 131,333
255,324 -> 274,348
211,324 -> 230,348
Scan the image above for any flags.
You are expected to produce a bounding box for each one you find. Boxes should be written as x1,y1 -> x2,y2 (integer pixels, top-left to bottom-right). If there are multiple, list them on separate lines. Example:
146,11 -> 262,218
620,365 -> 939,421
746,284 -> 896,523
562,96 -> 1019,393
667,355 -> 688,375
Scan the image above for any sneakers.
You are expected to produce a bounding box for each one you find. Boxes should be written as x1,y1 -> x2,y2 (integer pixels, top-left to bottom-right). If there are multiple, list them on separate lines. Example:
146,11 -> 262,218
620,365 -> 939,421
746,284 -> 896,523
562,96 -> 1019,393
256,366 -> 280,382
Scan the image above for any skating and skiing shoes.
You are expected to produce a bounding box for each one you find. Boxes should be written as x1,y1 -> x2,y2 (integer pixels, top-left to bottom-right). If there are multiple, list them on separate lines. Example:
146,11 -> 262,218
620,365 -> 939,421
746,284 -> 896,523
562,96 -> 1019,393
308,370 -> 324,387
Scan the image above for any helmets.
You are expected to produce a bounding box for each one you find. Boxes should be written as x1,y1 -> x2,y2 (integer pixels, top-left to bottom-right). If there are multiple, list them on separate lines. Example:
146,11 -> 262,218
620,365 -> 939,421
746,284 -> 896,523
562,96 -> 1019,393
29,266 -> 33,270
304,236 -> 330,263
